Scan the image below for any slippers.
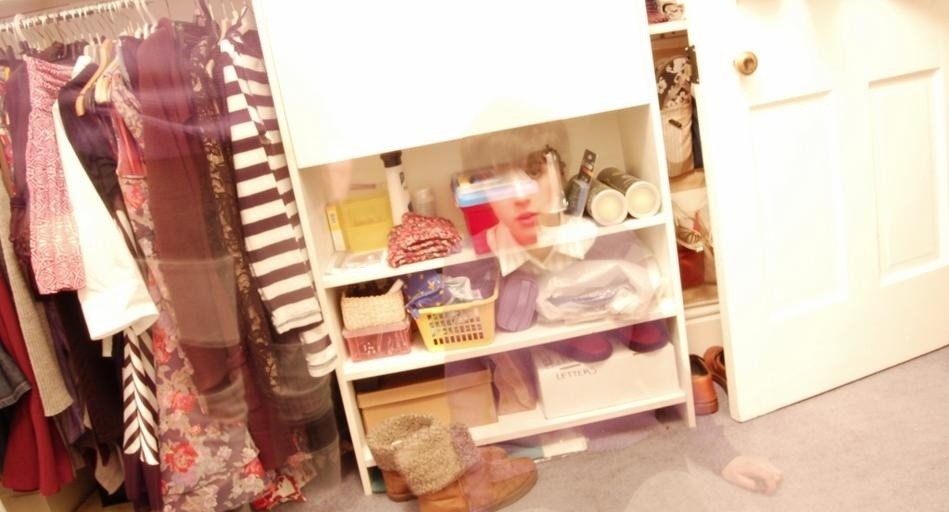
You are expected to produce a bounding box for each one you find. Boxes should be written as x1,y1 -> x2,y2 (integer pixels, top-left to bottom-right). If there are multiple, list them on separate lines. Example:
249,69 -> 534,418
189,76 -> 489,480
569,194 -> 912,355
545,332 -> 612,363
612,322 -> 668,351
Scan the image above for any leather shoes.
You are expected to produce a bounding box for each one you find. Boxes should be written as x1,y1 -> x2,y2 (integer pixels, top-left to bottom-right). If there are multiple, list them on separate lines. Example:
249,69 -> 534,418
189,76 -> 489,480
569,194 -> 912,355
705,346 -> 729,397
689,354 -> 718,415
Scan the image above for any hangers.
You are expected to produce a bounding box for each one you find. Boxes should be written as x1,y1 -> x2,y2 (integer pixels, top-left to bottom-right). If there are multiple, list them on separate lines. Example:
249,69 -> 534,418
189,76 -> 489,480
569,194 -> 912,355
0,0 -> 160,40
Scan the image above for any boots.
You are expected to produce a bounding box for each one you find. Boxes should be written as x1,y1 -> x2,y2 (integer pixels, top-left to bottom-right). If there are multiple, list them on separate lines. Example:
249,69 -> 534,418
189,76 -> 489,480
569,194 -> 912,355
395,421 -> 537,512
366,413 -> 508,502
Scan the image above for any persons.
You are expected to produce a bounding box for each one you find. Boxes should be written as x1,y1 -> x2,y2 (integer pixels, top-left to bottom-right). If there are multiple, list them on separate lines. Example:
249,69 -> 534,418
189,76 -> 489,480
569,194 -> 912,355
441,120 -> 784,512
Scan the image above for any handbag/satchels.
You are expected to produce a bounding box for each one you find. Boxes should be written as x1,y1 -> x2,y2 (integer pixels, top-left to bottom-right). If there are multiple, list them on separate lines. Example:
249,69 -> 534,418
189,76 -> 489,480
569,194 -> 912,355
497,272 -> 538,332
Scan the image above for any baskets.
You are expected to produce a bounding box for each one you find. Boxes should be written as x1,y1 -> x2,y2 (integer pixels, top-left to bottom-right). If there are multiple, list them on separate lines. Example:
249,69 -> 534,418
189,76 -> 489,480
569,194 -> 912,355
341,279 -> 405,330
405,271 -> 500,353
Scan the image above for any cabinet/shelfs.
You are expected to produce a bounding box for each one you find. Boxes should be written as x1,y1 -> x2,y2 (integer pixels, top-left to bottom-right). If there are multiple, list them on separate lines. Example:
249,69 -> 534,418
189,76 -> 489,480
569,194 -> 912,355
250,1 -> 696,497
646,1 -> 721,411
